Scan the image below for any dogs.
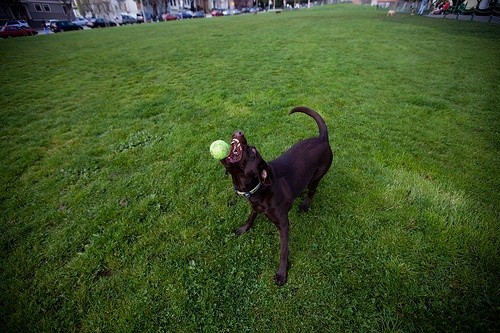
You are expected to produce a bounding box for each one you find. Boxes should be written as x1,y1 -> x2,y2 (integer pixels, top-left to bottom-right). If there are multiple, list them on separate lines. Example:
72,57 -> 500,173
387,10 -> 395,17
219,106 -> 333,288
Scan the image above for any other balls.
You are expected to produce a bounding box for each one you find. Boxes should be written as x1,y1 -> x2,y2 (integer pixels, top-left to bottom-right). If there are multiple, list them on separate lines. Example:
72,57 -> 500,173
209,140 -> 230,159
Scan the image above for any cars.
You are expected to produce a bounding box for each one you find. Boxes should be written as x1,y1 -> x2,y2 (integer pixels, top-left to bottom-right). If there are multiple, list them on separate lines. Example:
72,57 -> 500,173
210,6 -> 264,17
0,20 -> 39,38
86,10 -> 205,28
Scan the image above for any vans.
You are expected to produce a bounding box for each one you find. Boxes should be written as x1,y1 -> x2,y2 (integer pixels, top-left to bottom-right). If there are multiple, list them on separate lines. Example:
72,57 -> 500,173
45,17 -> 88,33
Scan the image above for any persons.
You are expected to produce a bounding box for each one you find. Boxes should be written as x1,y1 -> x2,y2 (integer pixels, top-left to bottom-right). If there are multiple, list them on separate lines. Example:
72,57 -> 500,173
40,8 -> 167,34
392,0 -> 491,16
